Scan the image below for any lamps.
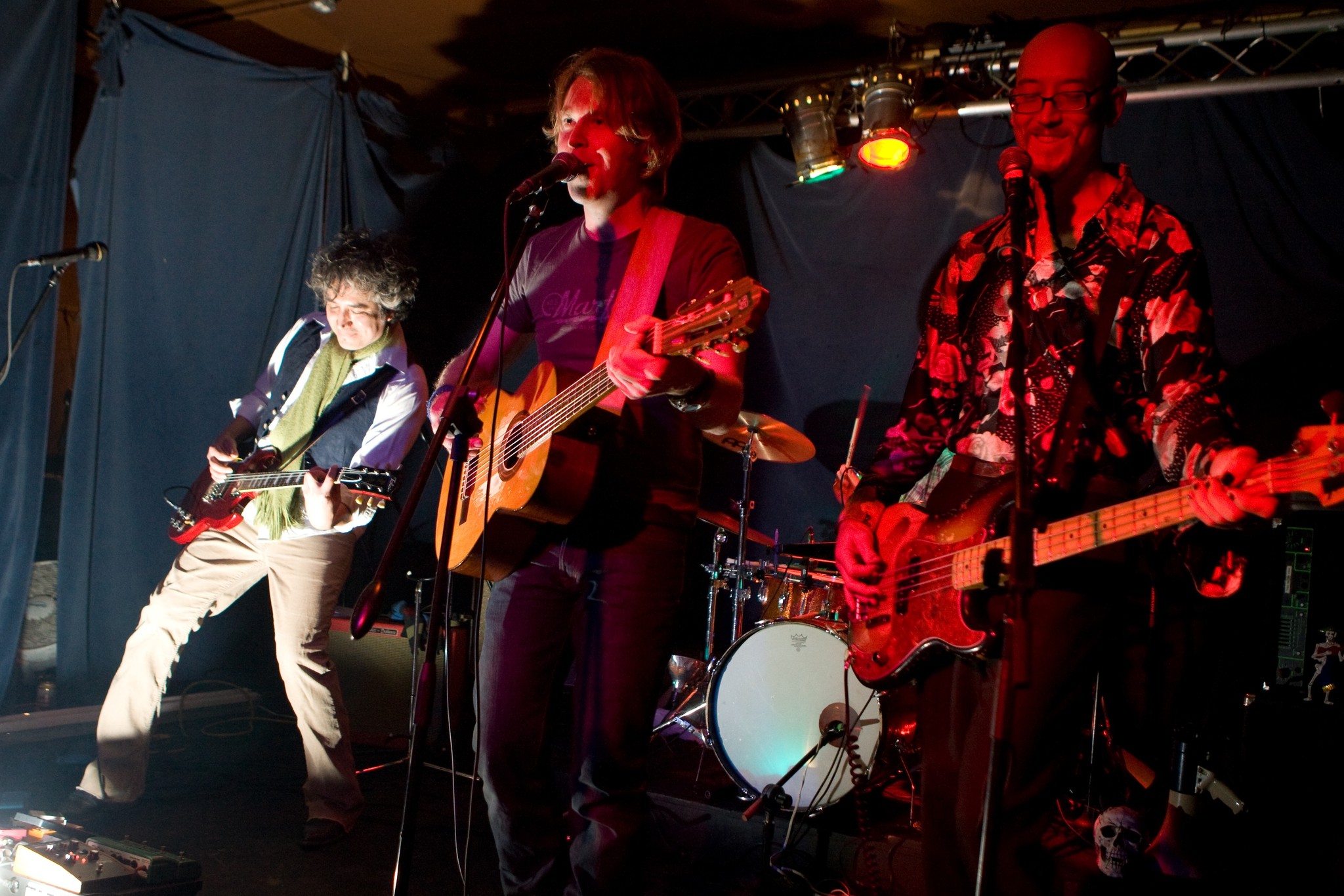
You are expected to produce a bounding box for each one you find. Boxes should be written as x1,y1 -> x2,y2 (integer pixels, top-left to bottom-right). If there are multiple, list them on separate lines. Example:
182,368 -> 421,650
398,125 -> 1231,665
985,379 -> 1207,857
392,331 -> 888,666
852,63 -> 918,170
765,80 -> 849,187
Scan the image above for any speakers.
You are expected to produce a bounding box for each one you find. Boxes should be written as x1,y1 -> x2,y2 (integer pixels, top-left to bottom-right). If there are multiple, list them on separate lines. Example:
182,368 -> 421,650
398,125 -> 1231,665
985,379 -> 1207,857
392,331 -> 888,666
324,614 -> 467,750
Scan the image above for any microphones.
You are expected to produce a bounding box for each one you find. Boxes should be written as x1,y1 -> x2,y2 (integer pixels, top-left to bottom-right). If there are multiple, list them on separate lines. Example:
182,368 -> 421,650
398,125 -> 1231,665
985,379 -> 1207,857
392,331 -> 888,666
996,146 -> 1032,206
19,240 -> 109,267
506,152 -> 580,204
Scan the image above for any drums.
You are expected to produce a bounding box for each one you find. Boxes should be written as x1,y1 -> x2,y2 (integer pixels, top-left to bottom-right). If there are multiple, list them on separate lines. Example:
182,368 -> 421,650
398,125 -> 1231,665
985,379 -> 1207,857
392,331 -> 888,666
705,611 -> 917,812
758,542 -> 850,629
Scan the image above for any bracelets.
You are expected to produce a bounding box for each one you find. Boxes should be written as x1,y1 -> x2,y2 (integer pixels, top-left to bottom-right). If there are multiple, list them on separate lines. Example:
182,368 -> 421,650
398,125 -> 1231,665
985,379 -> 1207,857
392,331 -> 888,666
427,384 -> 454,419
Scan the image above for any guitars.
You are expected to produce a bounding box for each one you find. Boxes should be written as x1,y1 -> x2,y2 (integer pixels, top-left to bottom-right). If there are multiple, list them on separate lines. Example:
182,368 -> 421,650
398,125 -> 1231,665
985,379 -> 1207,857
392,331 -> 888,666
168,445 -> 406,545
834,390 -> 1344,691
434,275 -> 770,581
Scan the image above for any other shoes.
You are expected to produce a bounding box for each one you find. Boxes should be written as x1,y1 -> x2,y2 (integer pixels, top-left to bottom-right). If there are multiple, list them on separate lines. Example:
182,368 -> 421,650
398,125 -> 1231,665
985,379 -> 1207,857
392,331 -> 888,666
57,791 -> 135,814
302,818 -> 344,848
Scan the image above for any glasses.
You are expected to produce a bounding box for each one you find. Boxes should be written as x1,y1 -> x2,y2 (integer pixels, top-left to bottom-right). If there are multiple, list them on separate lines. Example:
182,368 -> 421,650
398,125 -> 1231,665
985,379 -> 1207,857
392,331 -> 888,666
1007,82 -> 1111,116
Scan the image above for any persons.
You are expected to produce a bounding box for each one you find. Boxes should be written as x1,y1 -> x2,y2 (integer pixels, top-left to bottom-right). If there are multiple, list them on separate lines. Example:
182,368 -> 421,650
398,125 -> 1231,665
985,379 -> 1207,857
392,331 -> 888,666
427,48 -> 748,896
59,229 -> 428,848
832,24 -> 1278,896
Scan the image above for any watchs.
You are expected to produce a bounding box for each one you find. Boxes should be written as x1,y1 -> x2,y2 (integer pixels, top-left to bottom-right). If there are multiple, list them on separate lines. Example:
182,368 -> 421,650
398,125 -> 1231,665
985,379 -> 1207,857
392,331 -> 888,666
668,368 -> 716,413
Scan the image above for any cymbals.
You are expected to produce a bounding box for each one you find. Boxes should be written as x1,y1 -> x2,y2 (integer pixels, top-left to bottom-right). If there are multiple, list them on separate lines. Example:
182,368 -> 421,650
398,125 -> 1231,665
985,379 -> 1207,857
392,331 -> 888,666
695,509 -> 778,549
699,409 -> 816,462
804,398 -> 904,470
